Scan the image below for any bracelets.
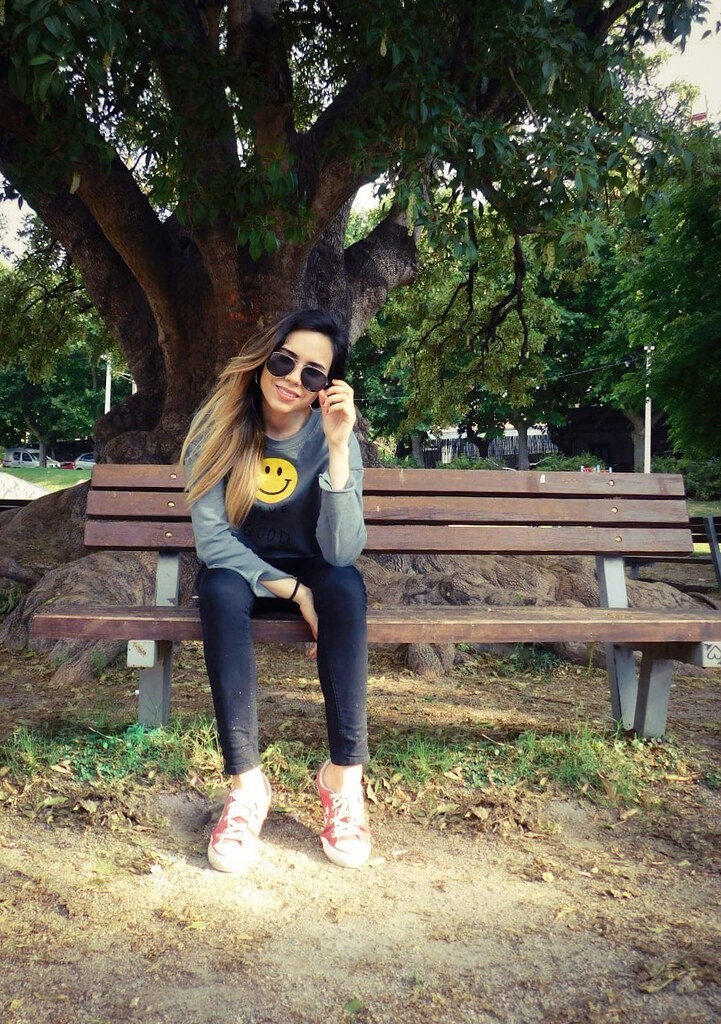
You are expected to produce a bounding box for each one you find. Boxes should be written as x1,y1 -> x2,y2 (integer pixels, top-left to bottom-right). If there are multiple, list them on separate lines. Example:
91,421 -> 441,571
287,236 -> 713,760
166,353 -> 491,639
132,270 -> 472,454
288,580 -> 299,601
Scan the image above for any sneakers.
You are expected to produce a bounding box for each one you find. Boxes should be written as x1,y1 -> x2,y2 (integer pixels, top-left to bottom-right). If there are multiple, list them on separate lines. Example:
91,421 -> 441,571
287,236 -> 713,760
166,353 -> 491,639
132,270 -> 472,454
207,773 -> 272,873
315,758 -> 372,870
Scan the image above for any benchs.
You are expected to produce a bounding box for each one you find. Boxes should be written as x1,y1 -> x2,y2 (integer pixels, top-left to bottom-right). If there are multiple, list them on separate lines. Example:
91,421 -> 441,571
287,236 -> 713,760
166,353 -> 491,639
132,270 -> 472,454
22,461 -> 721,740
622,515 -> 721,586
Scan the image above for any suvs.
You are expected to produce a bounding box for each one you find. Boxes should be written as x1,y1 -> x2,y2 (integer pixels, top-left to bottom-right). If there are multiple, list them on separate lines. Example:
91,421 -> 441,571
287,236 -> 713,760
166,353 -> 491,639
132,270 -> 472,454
73,452 -> 97,471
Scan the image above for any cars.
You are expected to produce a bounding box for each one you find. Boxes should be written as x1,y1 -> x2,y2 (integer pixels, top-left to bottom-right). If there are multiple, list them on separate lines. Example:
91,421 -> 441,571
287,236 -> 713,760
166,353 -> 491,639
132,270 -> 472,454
514,457 -> 556,471
59,461 -> 73,469
471,456 -> 517,471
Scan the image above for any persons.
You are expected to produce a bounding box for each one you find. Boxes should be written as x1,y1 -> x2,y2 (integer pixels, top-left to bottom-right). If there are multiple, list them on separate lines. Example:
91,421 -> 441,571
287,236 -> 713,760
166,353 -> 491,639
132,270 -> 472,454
180,310 -> 372,871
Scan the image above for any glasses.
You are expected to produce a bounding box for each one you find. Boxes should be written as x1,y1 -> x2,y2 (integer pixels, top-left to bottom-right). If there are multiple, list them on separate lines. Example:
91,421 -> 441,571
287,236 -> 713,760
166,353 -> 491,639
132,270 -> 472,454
265,351 -> 330,393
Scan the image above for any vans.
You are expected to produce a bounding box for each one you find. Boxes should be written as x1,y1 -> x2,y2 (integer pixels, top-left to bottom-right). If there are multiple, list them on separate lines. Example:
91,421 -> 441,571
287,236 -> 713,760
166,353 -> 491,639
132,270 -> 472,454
3,448 -> 61,468
